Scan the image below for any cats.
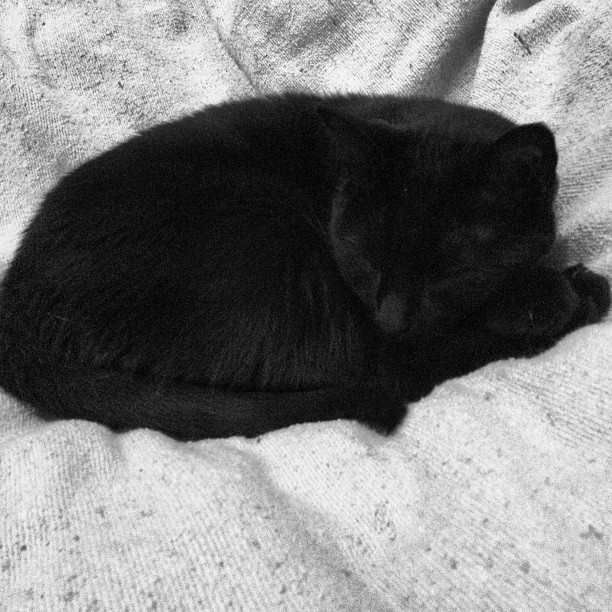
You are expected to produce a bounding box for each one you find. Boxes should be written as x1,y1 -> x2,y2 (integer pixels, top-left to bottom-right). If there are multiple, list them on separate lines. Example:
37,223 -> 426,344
0,86 -> 612,443
